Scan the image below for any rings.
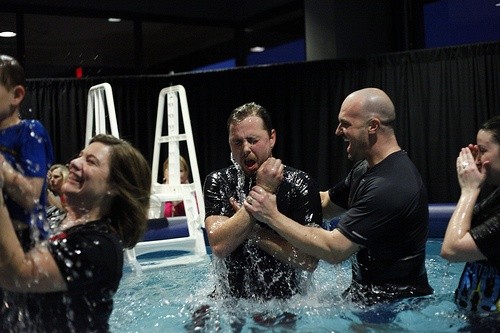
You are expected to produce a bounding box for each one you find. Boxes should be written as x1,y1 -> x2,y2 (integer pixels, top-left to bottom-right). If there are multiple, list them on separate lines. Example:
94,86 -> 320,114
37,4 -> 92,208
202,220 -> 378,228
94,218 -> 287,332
463,163 -> 469,168
247,198 -> 252,203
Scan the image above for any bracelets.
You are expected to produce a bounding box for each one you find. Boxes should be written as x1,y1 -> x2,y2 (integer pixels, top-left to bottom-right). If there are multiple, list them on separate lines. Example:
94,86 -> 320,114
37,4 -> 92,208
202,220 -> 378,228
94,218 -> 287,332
256,183 -> 273,193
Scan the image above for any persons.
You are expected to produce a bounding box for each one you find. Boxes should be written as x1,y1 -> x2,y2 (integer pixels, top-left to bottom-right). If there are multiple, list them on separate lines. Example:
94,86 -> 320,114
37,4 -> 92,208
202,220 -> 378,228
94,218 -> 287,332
244,88 -> 434,333
163,155 -> 200,219
184,102 -> 323,333
441,115 -> 500,333
0,54 -> 54,333
46,164 -> 70,210
0,134 -> 151,333
